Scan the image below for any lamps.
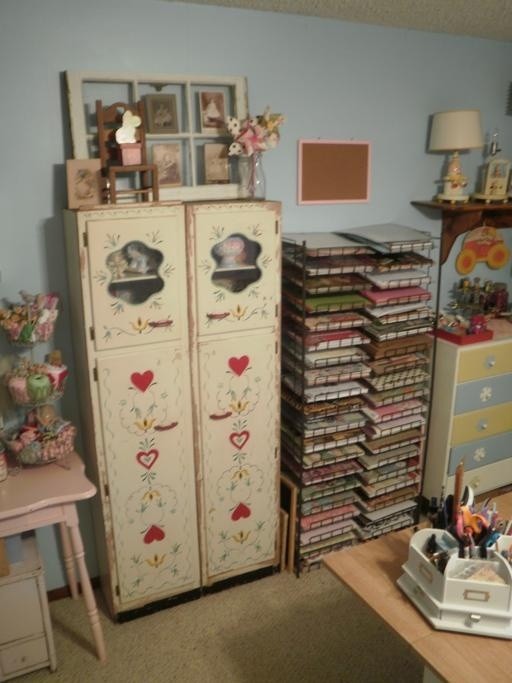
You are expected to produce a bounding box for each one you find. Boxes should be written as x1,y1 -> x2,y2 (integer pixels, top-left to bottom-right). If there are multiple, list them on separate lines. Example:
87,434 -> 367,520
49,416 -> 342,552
426,107 -> 484,202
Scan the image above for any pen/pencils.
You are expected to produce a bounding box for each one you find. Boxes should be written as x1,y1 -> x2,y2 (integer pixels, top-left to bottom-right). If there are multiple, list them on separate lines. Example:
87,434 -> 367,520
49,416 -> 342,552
426,532 -> 489,572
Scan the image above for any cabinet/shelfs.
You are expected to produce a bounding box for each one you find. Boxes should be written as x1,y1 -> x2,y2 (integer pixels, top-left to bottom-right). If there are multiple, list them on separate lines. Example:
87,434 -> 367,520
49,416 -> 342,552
421,318 -> 511,510
288,232 -> 440,574
0,537 -> 58,682
59,198 -> 287,620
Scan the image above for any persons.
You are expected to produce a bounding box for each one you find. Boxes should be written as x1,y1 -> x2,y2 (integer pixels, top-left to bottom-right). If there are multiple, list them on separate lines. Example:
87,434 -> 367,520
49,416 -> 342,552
204,97 -> 221,123
157,104 -> 169,126
161,154 -> 178,181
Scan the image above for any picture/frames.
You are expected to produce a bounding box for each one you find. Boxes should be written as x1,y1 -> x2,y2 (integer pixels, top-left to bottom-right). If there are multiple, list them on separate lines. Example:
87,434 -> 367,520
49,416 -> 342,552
295,140 -> 371,206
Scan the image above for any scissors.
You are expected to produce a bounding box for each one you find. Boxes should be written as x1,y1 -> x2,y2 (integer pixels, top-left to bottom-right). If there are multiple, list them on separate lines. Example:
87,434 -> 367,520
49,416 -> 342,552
464,515 -> 488,535
484,533 -> 501,547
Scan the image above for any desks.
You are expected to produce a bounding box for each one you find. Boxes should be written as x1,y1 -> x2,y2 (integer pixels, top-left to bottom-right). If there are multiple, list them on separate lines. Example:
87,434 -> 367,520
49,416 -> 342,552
323,492 -> 511,683
0,448 -> 107,678
